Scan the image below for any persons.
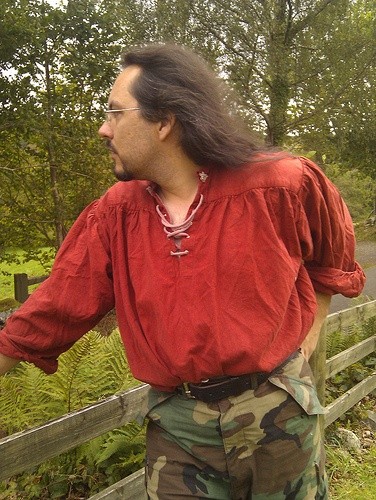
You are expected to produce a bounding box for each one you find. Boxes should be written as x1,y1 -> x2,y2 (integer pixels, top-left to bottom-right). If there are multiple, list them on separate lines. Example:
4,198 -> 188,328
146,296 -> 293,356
1,42 -> 365,500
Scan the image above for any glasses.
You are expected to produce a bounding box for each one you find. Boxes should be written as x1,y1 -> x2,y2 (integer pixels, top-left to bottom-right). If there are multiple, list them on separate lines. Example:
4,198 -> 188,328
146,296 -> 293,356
100,106 -> 143,122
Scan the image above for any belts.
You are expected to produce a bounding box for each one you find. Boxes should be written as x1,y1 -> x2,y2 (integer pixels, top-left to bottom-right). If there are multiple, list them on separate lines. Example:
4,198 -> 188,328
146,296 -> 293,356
174,372 -> 269,404
